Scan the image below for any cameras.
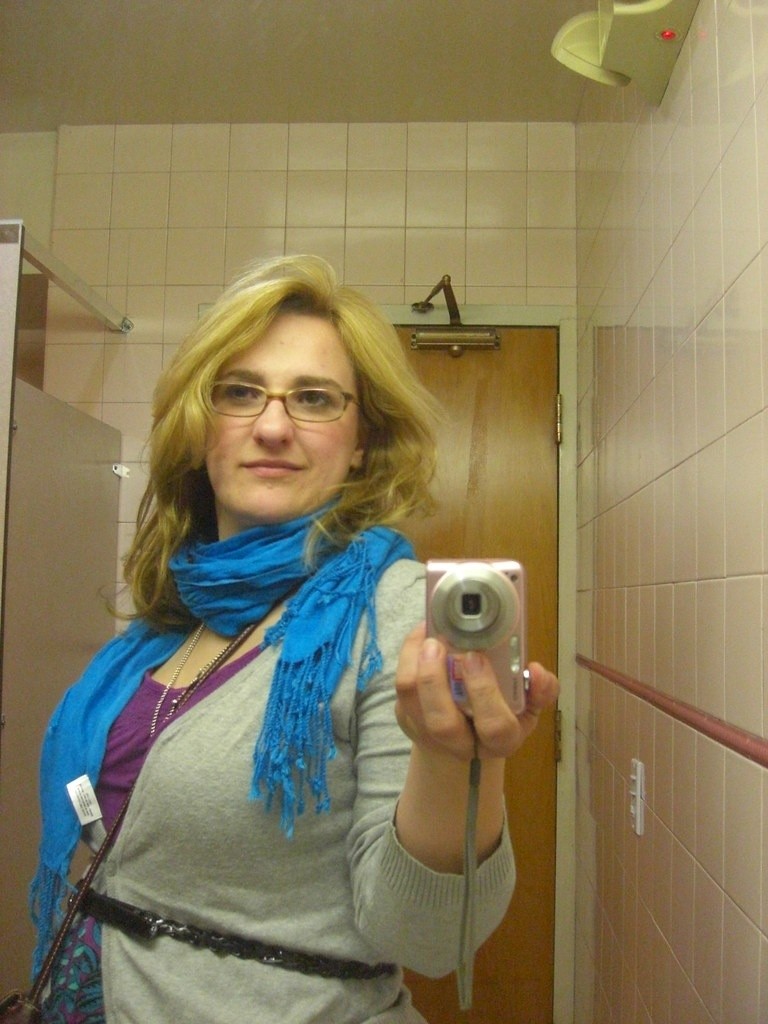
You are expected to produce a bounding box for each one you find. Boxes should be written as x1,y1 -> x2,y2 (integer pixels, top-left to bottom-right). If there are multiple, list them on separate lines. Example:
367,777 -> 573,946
426,557 -> 532,721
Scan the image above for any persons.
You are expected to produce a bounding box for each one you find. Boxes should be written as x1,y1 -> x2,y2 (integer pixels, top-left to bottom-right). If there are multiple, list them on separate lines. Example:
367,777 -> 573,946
27,248 -> 558,1024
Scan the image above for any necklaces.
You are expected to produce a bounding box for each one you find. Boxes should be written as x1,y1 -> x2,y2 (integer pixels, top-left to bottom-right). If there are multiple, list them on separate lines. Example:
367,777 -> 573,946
151,603 -> 261,737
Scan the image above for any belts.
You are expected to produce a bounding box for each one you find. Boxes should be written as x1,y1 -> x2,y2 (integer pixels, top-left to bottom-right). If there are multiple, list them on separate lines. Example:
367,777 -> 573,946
69,883 -> 399,982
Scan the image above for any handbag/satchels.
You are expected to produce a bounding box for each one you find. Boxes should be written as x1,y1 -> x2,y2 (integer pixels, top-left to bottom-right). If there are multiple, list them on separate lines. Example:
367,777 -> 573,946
0,987 -> 42,1024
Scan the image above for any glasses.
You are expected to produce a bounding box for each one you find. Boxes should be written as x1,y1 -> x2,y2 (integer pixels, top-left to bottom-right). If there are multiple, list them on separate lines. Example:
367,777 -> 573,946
209,381 -> 365,423
